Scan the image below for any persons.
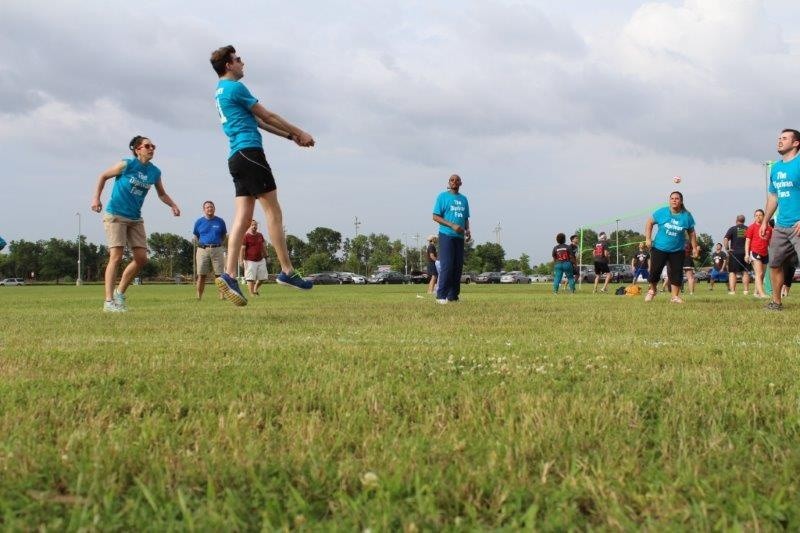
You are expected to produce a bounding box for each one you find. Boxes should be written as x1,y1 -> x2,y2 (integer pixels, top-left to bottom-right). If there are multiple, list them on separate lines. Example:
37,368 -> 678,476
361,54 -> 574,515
759,129 -> 800,311
427,235 -> 438,295
193,201 -> 227,300
240,220 -> 270,296
708,215 -> 753,295
210,46 -> 314,306
593,232 -> 612,293
436,175 -> 471,304
646,192 -> 699,304
552,233 -> 580,293
744,209 -> 773,297
92,135 -> 181,312
632,242 -> 651,285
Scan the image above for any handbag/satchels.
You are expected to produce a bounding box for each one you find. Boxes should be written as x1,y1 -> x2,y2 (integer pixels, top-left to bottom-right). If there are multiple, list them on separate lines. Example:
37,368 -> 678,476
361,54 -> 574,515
615,286 -> 625,295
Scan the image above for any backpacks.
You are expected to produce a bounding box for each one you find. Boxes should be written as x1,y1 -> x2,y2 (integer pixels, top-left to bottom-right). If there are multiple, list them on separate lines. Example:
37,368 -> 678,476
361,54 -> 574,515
625,285 -> 641,296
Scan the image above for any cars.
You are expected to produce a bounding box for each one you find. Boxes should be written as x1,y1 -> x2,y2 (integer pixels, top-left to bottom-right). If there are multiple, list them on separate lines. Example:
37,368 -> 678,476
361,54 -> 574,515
461,272 -> 471,284
374,271 -> 430,284
305,272 -> 368,285
476,271 -> 546,284
0,278 -> 24,286
583,264 -> 634,284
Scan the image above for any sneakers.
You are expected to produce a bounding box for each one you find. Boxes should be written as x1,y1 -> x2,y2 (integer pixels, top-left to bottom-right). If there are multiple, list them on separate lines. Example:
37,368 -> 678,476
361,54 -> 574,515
276,269 -> 314,290
215,273 -> 247,306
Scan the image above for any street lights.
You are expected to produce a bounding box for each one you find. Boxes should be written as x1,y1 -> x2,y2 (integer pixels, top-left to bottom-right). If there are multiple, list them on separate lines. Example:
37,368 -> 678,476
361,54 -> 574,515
616,217 -> 620,264
75,211 -> 82,286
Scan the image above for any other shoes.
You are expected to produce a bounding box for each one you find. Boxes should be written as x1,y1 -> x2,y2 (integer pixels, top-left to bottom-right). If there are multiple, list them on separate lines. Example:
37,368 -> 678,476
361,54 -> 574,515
112,289 -> 126,304
645,288 -> 658,301
728,290 -> 736,295
753,293 -> 770,299
744,290 -> 750,295
768,300 -> 783,311
103,301 -> 128,312
671,295 -> 685,302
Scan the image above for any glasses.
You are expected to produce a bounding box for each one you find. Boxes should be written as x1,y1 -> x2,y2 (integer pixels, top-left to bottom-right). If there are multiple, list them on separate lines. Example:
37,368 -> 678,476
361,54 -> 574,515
138,144 -> 156,151
234,56 -> 241,62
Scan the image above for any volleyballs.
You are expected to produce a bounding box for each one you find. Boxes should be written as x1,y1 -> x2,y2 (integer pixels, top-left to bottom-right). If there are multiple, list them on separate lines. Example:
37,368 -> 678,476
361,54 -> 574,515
672,175 -> 682,183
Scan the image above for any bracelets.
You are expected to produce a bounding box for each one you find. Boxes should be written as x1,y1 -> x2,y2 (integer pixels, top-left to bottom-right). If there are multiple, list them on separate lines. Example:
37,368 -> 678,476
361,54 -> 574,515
267,256 -> 269,257
287,133 -> 293,140
